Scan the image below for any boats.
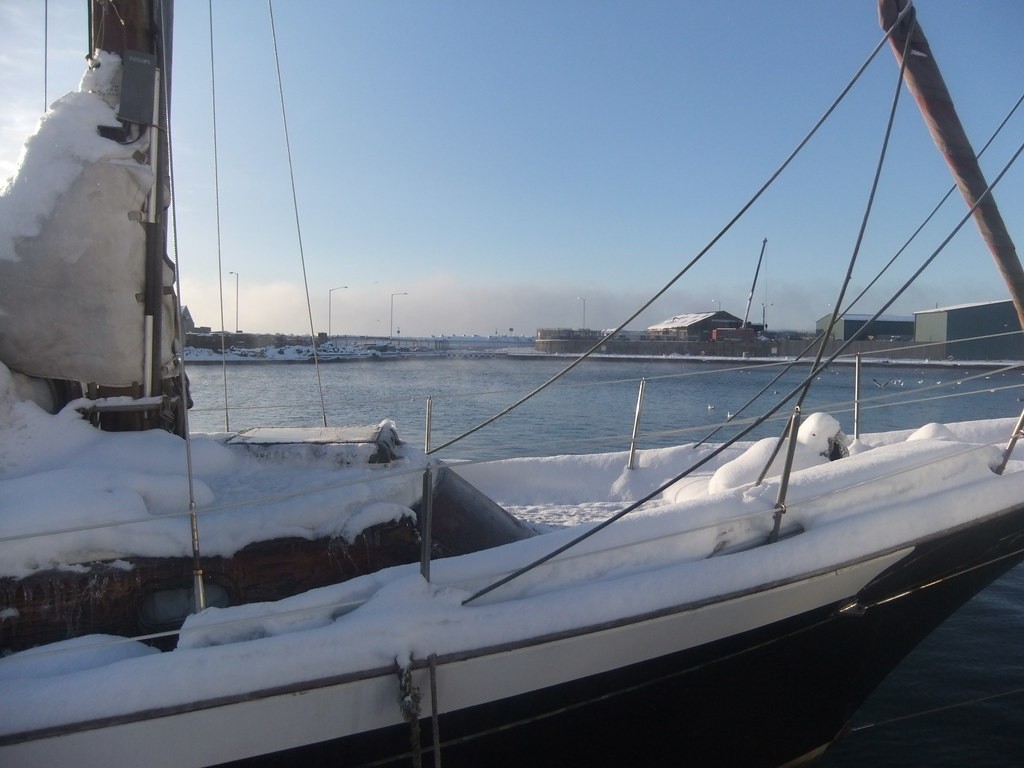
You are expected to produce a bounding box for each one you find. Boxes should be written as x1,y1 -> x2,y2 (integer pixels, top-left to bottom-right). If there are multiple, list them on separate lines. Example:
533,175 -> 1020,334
0,1 -> 1023,768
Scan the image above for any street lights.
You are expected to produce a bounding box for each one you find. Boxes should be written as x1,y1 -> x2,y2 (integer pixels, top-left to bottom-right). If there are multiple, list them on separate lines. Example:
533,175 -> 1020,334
329,287 -> 348,336
578,297 -> 585,329
712,300 -> 721,311
229,272 -> 239,333
390,293 -> 408,338
